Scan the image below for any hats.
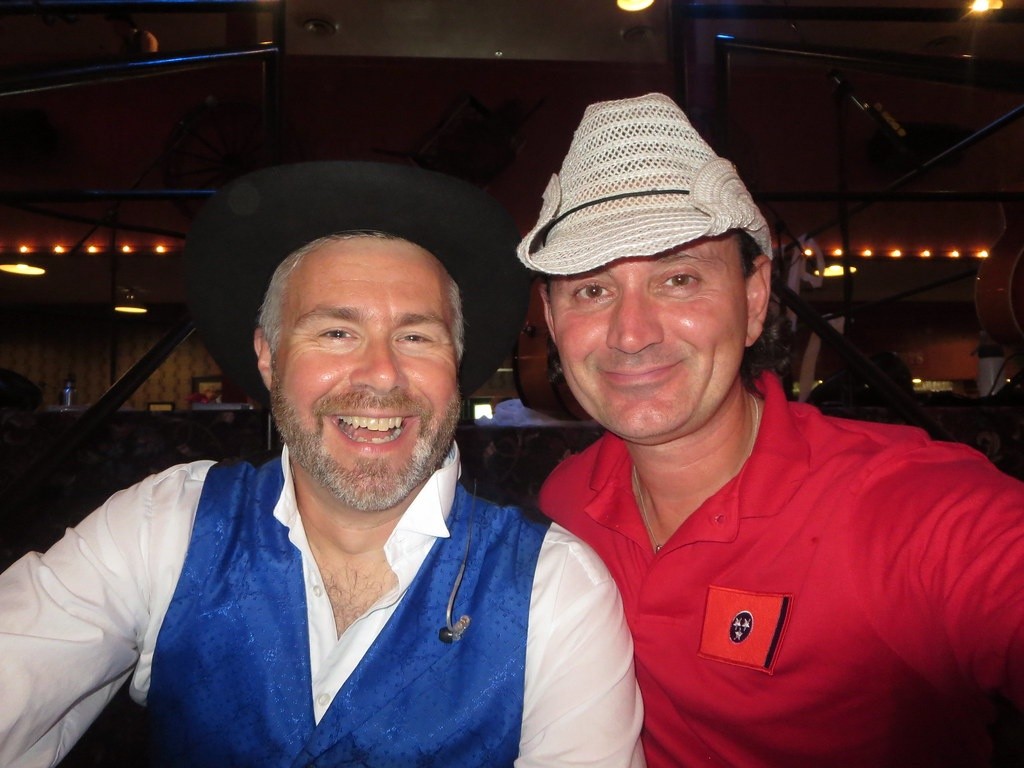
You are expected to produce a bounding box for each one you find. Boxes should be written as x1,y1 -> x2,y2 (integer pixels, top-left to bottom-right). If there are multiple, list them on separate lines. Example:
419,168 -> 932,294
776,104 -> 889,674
517,91 -> 774,277
175,142 -> 529,422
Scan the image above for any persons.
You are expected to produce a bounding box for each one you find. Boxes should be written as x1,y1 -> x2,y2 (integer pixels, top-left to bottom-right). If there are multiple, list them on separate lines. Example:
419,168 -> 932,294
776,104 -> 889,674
0,160 -> 647,768
515,91 -> 1024,768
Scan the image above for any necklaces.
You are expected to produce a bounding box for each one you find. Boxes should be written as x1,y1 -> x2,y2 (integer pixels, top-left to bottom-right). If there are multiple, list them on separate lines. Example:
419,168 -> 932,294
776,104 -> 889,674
634,393 -> 759,553
